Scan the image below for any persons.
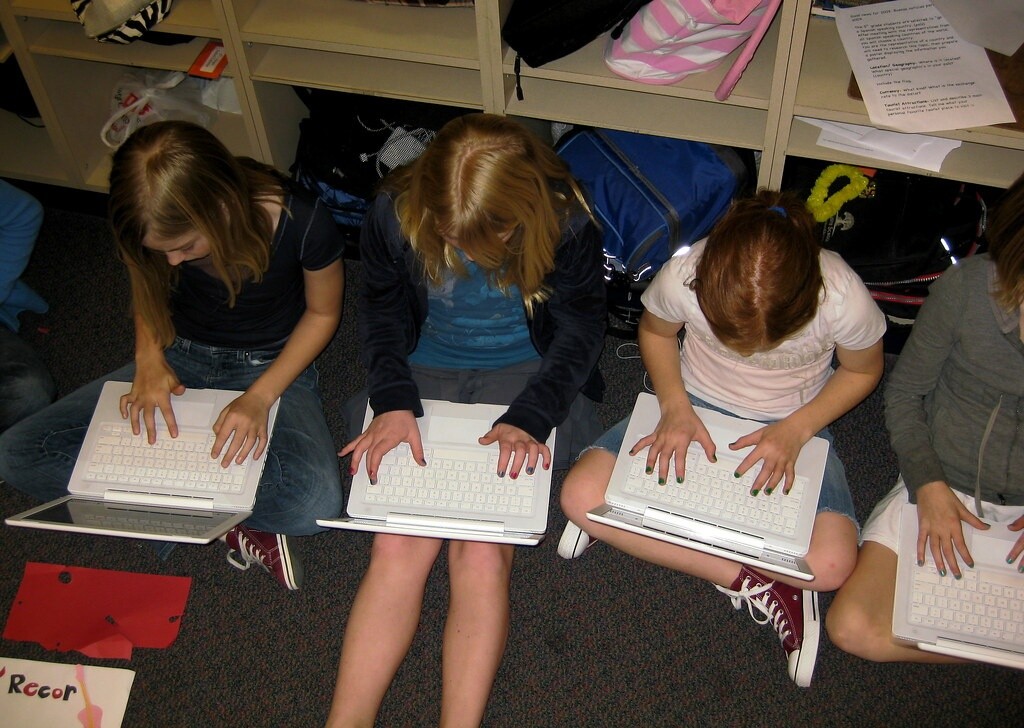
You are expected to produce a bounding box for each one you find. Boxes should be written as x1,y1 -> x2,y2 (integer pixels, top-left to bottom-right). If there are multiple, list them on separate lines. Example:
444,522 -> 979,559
558,208 -> 886,686
324,112 -> 611,728
0,120 -> 343,590
0,177 -> 54,427
827,177 -> 1024,664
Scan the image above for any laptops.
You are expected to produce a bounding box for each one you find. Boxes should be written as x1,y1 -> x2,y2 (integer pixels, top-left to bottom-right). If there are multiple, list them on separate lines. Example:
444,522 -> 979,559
890,503 -> 1024,670
5,381 -> 281,544
584,392 -> 829,581
315,398 -> 556,545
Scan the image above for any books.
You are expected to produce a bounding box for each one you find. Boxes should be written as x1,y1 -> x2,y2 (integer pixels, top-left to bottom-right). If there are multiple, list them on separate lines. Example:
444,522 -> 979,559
811,1 -> 835,19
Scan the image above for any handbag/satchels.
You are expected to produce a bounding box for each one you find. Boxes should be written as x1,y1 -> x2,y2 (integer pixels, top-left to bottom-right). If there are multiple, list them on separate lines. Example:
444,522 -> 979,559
606,1 -> 787,100
500,2 -> 651,99
70,0 -> 180,45
100,71 -> 219,148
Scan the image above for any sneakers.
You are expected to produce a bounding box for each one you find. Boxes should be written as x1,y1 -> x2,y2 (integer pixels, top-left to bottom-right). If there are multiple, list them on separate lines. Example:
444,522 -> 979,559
225,523 -> 304,592
718,563 -> 821,688
556,518 -> 598,561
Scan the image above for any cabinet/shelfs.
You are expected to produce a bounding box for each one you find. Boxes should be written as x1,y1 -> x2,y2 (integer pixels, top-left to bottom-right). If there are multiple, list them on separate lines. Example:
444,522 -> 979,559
0,0 -> 1024,250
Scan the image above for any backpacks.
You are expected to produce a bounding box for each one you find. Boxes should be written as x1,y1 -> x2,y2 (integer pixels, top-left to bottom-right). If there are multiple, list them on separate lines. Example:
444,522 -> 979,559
550,128 -> 734,300
799,160 -> 988,358
286,88 -> 454,237
